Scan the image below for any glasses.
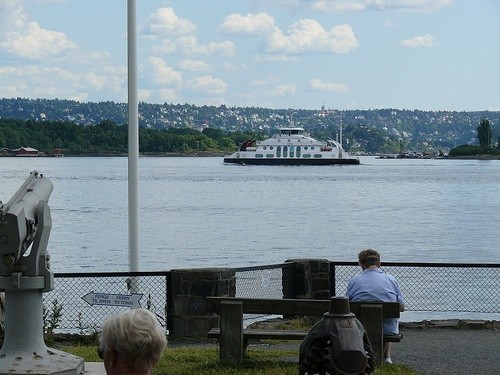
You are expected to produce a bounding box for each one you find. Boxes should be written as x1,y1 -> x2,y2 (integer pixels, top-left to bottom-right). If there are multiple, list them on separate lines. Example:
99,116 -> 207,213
97,348 -> 104,359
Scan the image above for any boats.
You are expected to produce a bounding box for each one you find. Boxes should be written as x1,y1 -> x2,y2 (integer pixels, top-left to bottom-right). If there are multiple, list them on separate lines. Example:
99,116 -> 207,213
223,114 -> 360,165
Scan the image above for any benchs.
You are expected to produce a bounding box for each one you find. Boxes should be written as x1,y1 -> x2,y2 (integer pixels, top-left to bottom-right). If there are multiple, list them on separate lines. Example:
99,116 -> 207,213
205,296 -> 403,367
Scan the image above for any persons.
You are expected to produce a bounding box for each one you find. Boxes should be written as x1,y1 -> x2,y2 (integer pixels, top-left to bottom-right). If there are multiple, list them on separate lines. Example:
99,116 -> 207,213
97,308 -> 166,375
346,248 -> 404,362
